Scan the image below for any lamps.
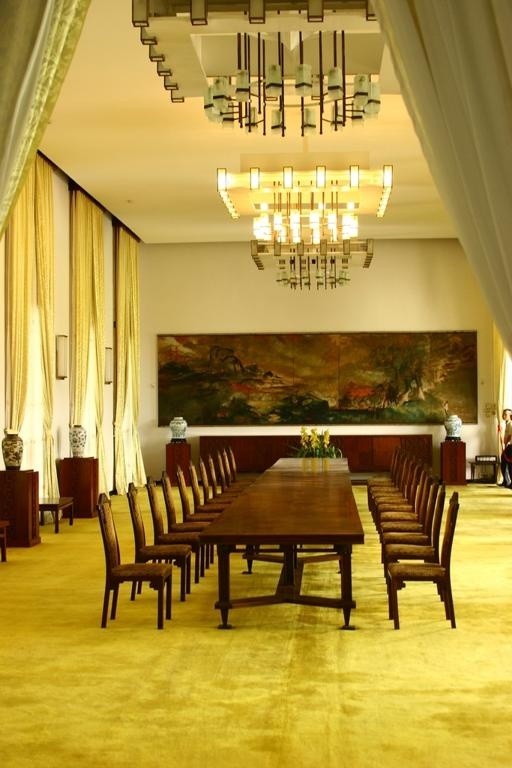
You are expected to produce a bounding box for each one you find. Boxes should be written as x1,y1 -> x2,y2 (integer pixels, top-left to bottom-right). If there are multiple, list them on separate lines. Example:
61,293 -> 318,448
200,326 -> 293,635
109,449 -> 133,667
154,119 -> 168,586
215,165 -> 396,294
55,335 -> 69,381
128,1 -> 387,137
104,346 -> 114,383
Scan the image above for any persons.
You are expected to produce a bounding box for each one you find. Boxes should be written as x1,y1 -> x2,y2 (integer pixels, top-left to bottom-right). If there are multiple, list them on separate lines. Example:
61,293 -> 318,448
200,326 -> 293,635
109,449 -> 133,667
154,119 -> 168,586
495,408 -> 511,490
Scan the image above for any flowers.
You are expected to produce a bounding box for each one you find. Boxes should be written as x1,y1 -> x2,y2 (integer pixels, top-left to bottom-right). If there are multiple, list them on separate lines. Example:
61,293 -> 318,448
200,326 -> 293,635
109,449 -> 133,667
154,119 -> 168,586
289,422 -> 341,459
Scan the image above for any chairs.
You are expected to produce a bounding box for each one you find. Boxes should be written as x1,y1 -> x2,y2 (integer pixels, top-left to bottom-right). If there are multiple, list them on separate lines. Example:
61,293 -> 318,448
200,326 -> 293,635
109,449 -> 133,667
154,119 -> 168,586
365,446 -> 462,632
95,441 -> 255,630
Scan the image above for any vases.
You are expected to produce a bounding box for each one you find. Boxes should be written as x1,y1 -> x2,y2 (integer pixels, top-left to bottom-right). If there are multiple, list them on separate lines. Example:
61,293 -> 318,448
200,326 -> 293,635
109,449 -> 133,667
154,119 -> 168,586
170,416 -> 188,443
68,424 -> 86,458
444,415 -> 462,439
308,453 -> 327,471
3,430 -> 24,471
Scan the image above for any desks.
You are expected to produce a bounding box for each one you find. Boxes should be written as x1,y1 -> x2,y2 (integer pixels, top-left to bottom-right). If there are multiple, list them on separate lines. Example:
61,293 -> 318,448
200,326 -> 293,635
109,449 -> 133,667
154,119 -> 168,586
38,494 -> 75,535
467,458 -> 500,484
199,455 -> 367,632
